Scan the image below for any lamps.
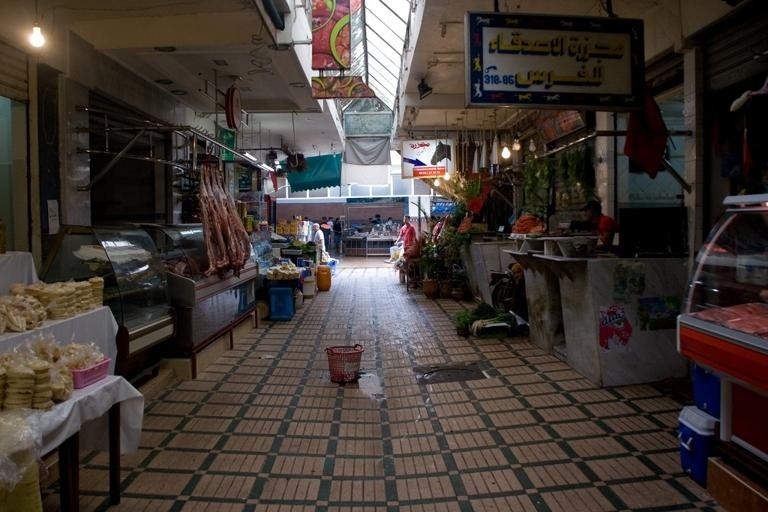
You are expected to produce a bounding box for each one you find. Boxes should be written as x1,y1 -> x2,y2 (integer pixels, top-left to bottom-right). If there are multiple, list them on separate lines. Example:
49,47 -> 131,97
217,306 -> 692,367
417,79 -> 435,100
434,107 -> 536,187
29,0 -> 45,47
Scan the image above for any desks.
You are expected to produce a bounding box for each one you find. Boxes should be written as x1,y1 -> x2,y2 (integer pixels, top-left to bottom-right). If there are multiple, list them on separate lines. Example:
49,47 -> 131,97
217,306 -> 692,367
0,251 -> 145,512
407,257 -> 444,292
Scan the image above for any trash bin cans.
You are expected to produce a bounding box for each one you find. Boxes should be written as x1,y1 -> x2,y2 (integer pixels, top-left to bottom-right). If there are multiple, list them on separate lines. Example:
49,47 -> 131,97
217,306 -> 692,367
325,343 -> 364,384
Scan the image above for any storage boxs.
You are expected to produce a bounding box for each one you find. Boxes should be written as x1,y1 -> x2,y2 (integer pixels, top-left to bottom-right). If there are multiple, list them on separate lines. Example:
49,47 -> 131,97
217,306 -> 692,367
690,360 -> 721,418
677,406 -> 719,488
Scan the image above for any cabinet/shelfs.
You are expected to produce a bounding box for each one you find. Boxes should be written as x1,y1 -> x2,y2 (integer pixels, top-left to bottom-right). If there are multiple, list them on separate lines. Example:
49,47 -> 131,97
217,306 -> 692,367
677,194 -> 768,489
40,224 -> 176,377
137,223 -> 258,379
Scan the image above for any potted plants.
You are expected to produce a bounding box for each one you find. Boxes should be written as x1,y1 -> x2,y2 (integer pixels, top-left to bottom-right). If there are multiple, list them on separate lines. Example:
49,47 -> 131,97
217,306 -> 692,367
421,230 -> 470,301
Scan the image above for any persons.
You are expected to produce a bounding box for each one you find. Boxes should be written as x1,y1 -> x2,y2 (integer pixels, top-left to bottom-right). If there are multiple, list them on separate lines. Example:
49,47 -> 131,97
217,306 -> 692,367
431,215 -> 445,242
511,262 -> 528,322
368,214 -> 392,230
394,216 -> 416,251
313,216 -> 342,252
